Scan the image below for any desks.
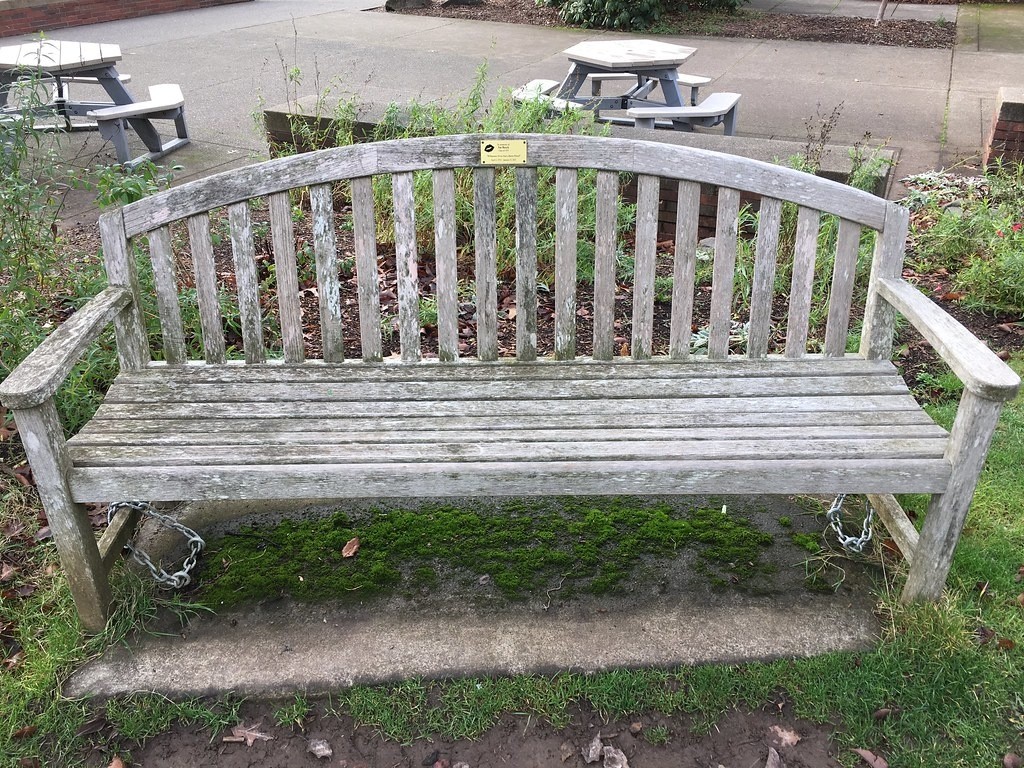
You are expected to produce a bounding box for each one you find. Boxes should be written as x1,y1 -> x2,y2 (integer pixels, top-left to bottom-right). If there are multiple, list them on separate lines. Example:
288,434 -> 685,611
0,39 -> 123,132
562,38 -> 697,133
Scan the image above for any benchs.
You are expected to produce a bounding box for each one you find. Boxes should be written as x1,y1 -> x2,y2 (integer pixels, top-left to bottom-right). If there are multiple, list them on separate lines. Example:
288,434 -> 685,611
587,72 -> 711,107
1,133 -> 1019,635
629,92 -> 743,136
514,77 -> 582,120
87,83 -> 191,172
11,73 -> 133,105
